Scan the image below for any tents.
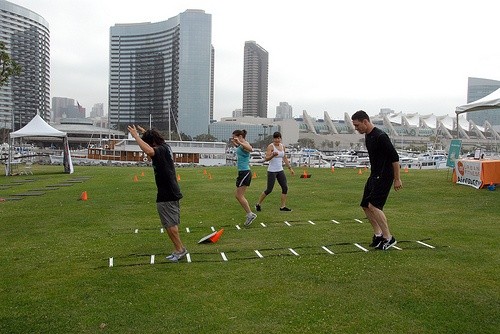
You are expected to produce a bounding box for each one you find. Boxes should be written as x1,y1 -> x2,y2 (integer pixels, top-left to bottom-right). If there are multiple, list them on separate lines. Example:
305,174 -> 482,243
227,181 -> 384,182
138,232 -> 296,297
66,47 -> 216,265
5,112 -> 71,178
455,84 -> 500,168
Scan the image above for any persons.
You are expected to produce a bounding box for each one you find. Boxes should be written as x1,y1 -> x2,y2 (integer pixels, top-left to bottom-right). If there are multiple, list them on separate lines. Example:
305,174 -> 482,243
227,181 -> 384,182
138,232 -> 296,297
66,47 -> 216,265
351,110 -> 403,251
252,132 -> 295,212
229,129 -> 258,227
126,122 -> 190,261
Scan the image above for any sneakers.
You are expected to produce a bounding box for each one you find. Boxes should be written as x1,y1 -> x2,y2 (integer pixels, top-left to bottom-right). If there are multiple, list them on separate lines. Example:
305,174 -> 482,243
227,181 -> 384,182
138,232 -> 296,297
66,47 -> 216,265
368,234 -> 383,248
375,236 -> 397,251
255,203 -> 261,212
168,247 -> 188,261
280,207 -> 291,211
166,251 -> 175,259
244,213 -> 257,226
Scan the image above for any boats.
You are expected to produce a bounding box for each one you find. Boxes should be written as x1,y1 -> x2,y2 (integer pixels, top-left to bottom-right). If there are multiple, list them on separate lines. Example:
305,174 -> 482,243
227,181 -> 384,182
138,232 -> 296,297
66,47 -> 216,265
226,145 -> 451,169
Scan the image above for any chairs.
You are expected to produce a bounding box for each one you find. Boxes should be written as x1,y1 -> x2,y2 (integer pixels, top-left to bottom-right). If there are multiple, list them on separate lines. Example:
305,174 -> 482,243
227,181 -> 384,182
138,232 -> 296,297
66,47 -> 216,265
23,162 -> 34,176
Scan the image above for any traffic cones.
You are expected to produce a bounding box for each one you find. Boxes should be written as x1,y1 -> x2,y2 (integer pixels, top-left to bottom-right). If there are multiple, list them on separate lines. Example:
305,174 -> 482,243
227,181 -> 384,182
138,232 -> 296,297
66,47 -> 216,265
358,169 -> 362,175
177,174 -> 181,182
404,166 -> 409,173
203,168 -> 207,175
252,172 -> 256,179
300,170 -> 312,179
207,173 -> 212,180
209,228 -> 224,243
80,191 -> 88,201
331,166 -> 335,172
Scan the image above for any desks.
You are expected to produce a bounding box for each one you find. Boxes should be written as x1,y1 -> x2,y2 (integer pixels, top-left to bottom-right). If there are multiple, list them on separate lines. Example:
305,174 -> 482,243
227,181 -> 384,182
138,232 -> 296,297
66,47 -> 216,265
454,159 -> 500,190
2,162 -> 21,176
